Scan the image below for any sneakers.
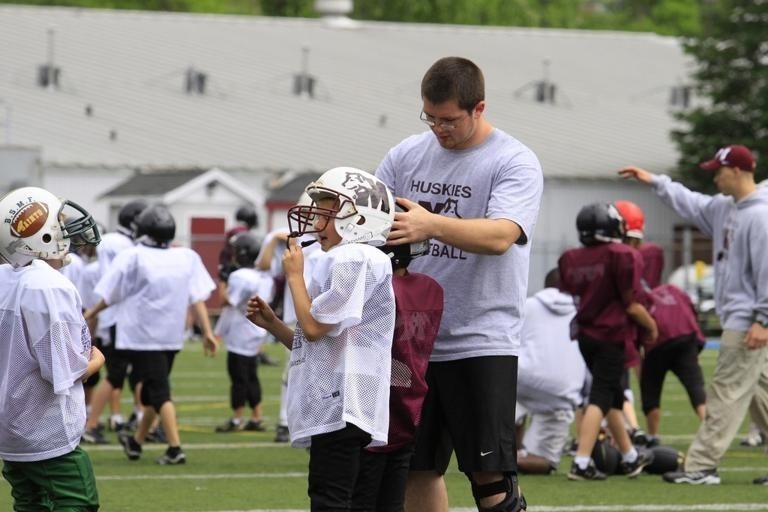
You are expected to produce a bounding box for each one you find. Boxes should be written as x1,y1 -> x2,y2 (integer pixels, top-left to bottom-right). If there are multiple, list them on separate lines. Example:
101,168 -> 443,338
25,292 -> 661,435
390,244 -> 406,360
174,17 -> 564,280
157,446 -> 187,465
647,436 -> 660,449
243,420 -> 268,431
109,420 -> 125,432
620,448 -> 654,478
118,432 -> 142,460
631,428 -> 647,445
567,459 -> 608,481
740,432 -> 765,446
274,425 -> 289,442
663,468 -> 721,485
82,428 -> 110,444
215,420 -> 242,432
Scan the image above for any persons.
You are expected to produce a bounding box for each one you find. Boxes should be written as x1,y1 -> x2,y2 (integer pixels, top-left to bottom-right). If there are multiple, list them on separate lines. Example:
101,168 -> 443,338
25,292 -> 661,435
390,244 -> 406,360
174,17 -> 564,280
558,201 -> 658,481
368,56 -> 543,511
78,198 -> 148,443
85,203 -> 217,463
625,242 -> 711,446
513,267 -> 592,473
619,145 -> 767,487
215,236 -> 271,432
610,201 -> 656,445
0,186 -> 104,511
244,165 -> 395,512
219,210 -> 276,367
367,201 -> 443,510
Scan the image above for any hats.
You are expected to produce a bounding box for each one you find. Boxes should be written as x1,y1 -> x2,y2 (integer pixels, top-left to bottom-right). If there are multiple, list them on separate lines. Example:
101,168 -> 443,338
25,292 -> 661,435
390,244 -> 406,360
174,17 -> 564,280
700,145 -> 757,173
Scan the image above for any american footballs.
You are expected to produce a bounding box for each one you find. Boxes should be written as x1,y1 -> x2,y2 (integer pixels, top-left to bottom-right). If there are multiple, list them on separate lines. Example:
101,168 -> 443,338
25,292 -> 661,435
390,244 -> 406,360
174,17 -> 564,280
642,446 -> 686,475
591,433 -> 622,475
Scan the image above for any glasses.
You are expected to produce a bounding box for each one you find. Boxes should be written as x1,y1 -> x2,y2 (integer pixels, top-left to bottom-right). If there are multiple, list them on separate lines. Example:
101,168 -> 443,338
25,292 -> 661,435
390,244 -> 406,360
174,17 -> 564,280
419,107 -> 469,132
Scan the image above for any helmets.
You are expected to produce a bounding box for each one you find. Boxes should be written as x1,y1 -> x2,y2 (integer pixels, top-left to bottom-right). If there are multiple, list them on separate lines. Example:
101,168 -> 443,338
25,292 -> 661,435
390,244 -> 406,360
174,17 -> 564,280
613,200 -> 645,240
0,187 -> 102,269
287,167 -> 395,253
576,203 -> 628,246
229,231 -> 260,267
236,208 -> 258,230
376,201 -> 430,269
133,205 -> 176,249
116,202 -> 149,239
